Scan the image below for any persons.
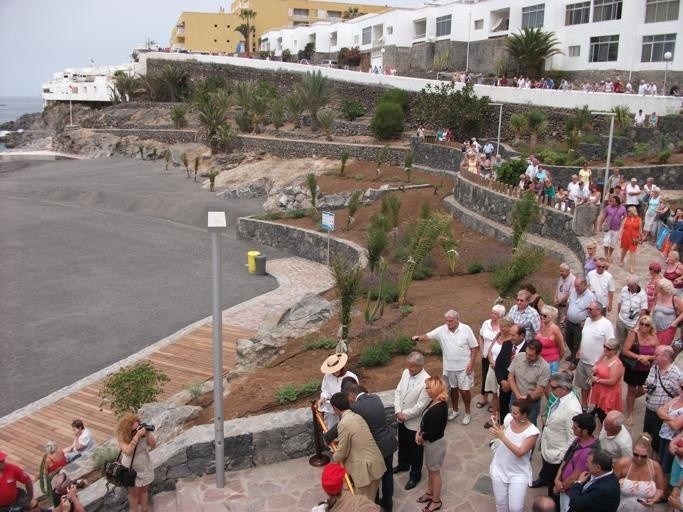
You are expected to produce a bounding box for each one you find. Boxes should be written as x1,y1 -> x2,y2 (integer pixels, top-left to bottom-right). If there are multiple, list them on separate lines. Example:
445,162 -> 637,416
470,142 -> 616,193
117,412 -> 157,512
391,351 -> 447,512
0,414 -> 96,512
519,156 -> 683,272
310,353 -> 397,512
479,243 -> 683,511
450,70 -> 470,83
146,44 -> 309,65
512,75 -> 681,96
634,109 -> 644,128
477,73 -> 508,87
647,111 -> 661,130
463,137 -> 504,181
412,310 -> 479,425
416,125 -> 454,142
368,65 -> 396,76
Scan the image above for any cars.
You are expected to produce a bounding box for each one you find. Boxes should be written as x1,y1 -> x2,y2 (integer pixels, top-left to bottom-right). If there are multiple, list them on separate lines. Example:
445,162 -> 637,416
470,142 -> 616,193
249,51 -> 277,61
299,59 -> 316,67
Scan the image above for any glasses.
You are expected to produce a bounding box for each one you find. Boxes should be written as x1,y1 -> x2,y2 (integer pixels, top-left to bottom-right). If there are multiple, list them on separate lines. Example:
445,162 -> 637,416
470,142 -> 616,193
585,307 -> 601,312
60,471 -> 68,488
603,344 -> 619,352
549,383 -> 564,391
632,451 -> 648,459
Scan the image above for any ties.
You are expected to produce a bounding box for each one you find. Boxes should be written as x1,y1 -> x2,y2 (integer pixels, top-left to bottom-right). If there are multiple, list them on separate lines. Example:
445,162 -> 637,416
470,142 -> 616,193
508,345 -> 517,365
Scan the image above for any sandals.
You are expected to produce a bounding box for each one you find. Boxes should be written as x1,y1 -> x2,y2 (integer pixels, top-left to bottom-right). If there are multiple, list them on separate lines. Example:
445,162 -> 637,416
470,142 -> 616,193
420,499 -> 442,512
416,491 -> 434,504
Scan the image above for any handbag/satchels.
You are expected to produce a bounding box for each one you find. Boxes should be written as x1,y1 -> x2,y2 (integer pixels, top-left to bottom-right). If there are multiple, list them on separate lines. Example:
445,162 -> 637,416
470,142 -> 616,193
599,220 -> 612,232
675,314 -> 683,328
619,341 -> 641,373
103,462 -> 139,488
631,237 -> 641,246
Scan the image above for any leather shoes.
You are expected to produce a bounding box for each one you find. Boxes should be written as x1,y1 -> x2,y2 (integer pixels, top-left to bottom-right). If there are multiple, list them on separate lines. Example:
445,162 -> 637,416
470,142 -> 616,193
403,472 -> 423,491
393,462 -> 411,475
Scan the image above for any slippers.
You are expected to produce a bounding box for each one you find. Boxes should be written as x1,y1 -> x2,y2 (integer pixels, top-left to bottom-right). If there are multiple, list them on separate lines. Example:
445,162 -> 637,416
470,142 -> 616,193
475,400 -> 502,450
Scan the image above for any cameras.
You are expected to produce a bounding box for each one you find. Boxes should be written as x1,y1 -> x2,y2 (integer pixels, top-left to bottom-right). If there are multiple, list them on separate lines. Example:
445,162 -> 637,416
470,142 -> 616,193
71,480 -> 84,489
629,308 -> 636,318
137,423 -> 154,437
646,384 -> 656,393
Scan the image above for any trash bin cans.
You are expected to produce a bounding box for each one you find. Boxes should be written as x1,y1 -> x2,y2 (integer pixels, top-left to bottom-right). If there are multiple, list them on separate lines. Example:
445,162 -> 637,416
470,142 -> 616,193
247,251 -> 266,275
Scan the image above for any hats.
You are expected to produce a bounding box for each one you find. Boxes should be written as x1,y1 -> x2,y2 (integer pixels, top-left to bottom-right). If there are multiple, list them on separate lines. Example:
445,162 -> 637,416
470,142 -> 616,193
319,352 -> 350,375
321,463 -> 347,495
0,450 -> 8,464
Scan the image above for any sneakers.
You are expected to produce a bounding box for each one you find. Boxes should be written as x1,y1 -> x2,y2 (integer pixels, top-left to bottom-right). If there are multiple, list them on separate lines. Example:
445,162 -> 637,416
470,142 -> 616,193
568,362 -> 576,371
447,410 -> 459,422
625,415 -> 635,429
565,354 -> 573,362
461,412 -> 472,425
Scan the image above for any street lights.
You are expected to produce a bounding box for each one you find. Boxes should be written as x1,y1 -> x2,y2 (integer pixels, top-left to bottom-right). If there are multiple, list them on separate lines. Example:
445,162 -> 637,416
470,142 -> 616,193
280,43 -> 283,61
589,111 -> 617,202
487,103 -> 503,155
663,51 -> 673,97
327,35 -> 333,54
379,48 -> 385,73
205,207 -> 231,484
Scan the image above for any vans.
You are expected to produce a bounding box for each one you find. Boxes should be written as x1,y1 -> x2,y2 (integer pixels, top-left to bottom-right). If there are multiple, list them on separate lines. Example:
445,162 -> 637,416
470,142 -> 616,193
316,59 -> 338,68
